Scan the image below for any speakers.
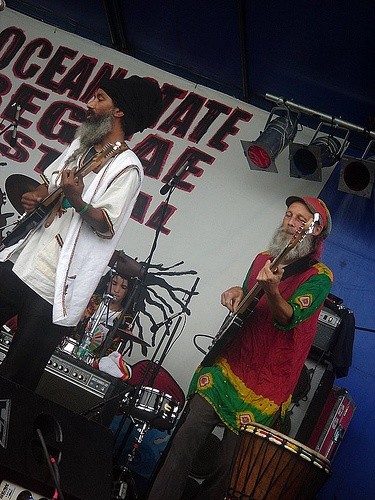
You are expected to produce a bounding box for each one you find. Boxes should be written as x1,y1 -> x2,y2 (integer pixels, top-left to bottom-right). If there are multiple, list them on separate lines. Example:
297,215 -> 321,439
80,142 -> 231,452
279,357 -> 334,442
0,375 -> 113,500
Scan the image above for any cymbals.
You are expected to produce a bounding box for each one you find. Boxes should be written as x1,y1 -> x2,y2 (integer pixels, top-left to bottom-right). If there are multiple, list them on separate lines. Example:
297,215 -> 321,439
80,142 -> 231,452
100,322 -> 151,347
107,249 -> 146,276
5,174 -> 42,217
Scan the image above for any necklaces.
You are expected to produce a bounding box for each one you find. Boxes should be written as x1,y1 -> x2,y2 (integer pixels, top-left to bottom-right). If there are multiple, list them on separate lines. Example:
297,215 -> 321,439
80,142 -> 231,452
91,300 -> 124,328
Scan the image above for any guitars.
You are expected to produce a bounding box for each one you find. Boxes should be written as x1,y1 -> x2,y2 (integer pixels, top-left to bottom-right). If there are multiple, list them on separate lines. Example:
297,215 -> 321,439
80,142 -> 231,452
200,212 -> 320,367
1,141 -> 127,247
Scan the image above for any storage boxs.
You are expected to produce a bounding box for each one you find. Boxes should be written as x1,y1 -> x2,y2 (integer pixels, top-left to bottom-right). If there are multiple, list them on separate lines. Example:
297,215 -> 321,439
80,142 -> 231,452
307,383 -> 358,463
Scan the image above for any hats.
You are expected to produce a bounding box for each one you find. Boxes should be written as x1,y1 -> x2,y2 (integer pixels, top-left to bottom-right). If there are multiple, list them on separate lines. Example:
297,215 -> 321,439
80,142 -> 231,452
285,195 -> 332,241
97,75 -> 162,135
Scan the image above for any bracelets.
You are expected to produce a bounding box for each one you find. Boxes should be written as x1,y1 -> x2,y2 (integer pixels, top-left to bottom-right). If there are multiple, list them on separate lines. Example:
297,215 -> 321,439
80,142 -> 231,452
75,202 -> 89,215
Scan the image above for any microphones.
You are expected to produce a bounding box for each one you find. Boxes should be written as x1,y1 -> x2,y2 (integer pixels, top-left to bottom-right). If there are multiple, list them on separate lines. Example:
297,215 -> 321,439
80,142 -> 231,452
160,157 -> 193,194
9,103 -> 20,147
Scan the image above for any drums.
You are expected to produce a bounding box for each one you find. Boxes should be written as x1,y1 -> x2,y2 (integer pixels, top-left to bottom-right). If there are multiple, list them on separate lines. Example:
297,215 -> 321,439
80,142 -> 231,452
122,385 -> 182,432
56,336 -> 96,366
223,422 -> 332,500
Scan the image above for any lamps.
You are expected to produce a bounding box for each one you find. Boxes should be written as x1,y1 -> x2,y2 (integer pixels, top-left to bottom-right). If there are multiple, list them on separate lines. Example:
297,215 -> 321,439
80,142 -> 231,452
288,121 -> 350,182
337,139 -> 375,198
239,104 -> 303,174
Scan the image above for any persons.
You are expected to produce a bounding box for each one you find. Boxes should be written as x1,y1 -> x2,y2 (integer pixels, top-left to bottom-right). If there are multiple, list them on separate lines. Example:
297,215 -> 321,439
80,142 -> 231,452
149,196 -> 334,500
0,75 -> 163,451
64,268 -> 135,369
